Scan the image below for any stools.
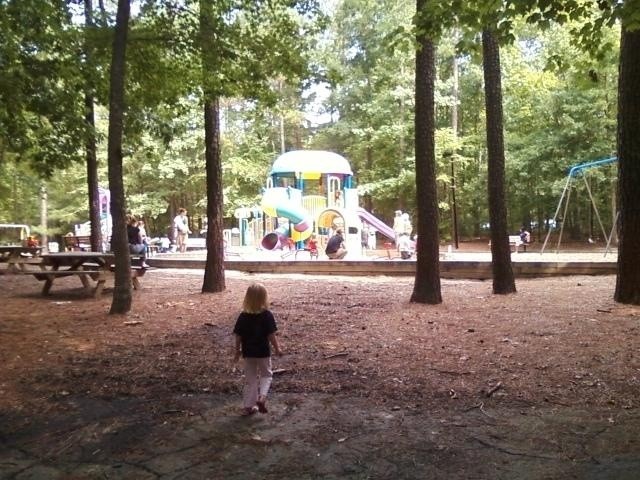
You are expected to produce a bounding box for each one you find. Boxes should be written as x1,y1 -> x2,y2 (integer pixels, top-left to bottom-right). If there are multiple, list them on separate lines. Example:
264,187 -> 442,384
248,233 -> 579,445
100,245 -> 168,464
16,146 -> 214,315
147,246 -> 158,257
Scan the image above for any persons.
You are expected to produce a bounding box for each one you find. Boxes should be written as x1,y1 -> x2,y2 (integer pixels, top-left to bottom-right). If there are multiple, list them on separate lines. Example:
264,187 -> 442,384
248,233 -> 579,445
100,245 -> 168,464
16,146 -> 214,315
523,230 -> 531,252
16,207 -> 208,268
513,226 -> 526,253
305,189 -> 419,261
232,283 -> 284,416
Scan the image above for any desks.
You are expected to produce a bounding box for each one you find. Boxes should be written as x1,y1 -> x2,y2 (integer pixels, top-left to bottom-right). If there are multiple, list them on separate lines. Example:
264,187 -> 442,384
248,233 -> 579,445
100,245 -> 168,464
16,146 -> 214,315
488,236 -> 529,252
39,253 -> 142,299
0,247 -> 45,273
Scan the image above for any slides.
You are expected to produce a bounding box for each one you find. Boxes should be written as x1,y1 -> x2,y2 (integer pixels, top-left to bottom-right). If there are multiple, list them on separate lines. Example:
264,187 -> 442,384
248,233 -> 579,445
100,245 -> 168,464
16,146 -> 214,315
359,208 -> 417,248
261,187 -> 313,251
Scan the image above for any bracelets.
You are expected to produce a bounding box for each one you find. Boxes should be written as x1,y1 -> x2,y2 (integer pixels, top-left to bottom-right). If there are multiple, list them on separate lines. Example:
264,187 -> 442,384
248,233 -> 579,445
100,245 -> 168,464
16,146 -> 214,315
236,345 -> 240,350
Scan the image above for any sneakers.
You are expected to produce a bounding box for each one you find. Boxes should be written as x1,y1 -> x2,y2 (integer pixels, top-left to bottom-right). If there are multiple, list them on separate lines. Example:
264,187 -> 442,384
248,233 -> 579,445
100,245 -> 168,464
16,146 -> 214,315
240,398 -> 269,416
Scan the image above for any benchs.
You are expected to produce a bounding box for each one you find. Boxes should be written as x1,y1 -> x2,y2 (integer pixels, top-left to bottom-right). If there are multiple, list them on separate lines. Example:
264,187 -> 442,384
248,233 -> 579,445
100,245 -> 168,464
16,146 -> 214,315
0,256 -> 40,274
24,263 -> 156,299
65,236 -> 91,251
